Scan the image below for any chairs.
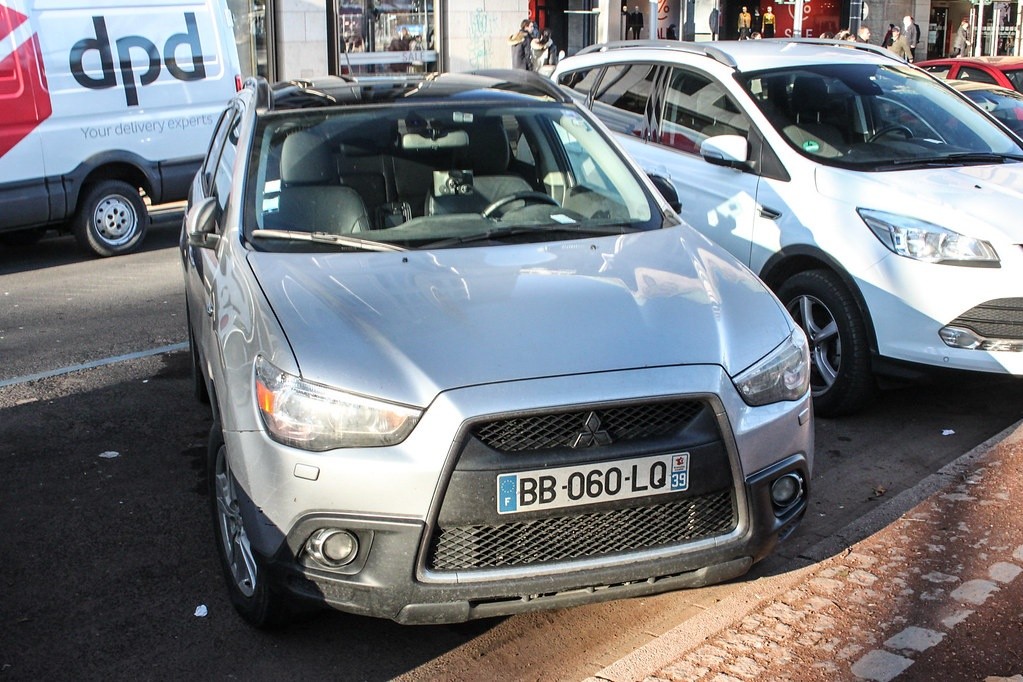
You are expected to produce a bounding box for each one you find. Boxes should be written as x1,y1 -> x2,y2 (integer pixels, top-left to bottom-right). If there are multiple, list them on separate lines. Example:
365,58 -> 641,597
782,73 -> 847,158
263,131 -> 374,233
692,88 -> 740,152
429,119 -> 536,217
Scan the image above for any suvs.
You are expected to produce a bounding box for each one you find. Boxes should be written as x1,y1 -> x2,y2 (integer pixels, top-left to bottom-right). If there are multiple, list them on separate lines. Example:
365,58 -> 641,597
516,37 -> 1023,425
177,67 -> 815,645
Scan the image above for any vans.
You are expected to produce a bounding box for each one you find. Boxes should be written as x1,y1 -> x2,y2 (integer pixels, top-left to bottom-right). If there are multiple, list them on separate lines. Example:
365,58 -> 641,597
1,0 -> 242,259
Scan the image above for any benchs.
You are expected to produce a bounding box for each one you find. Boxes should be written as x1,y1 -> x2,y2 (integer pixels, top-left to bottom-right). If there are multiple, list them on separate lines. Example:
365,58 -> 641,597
331,118 -> 433,219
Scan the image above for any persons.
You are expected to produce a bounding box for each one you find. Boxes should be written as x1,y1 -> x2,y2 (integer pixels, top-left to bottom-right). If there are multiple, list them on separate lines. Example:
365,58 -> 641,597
508,19 -> 558,72
751,6 -> 762,32
761,6 -> 776,38
737,6 -> 751,41
949,20 -> 971,58
880,15 -> 921,64
666,24 -> 677,40
388,26 -> 425,73
621,5 -> 644,40
819,25 -> 870,52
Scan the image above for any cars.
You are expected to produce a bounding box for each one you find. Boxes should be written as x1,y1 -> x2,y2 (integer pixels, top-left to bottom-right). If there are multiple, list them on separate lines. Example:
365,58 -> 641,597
870,55 -> 1023,148
